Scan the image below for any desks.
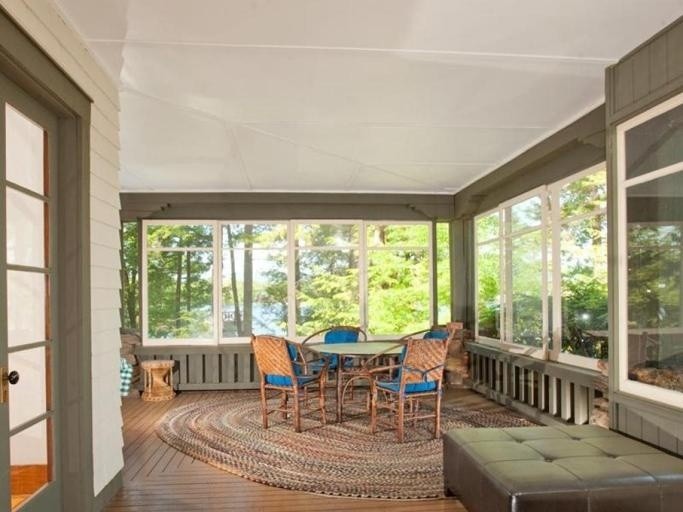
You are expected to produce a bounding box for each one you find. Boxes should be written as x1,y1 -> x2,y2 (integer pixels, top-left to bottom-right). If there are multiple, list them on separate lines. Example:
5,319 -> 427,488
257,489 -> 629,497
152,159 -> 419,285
309,343 -> 407,433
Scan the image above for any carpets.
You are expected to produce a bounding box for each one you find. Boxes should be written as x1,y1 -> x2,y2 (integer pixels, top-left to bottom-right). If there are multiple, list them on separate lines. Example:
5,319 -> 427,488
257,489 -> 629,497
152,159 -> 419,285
155,388 -> 534,499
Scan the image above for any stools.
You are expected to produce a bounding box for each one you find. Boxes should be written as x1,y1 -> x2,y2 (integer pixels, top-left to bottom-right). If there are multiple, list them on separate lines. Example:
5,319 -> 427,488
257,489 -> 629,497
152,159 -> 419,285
140,359 -> 177,401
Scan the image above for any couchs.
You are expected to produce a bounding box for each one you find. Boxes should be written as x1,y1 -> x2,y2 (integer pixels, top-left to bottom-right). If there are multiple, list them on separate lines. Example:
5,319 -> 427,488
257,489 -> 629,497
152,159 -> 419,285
443,424 -> 683,512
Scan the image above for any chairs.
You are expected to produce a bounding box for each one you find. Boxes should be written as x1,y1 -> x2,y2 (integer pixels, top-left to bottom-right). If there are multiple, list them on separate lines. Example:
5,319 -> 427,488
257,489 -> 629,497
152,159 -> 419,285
300,327 -> 364,399
251,335 -> 328,433
373,338 -> 447,442
399,325 -> 454,407
